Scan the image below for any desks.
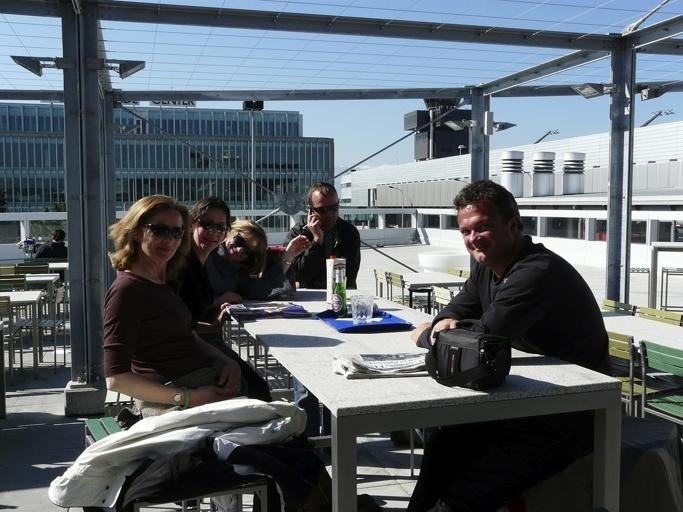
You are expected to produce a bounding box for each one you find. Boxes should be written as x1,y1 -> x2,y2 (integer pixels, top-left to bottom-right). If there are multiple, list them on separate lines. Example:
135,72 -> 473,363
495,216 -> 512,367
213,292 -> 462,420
649,241 -> 683,309
224,287 -> 622,512
598,308 -> 683,358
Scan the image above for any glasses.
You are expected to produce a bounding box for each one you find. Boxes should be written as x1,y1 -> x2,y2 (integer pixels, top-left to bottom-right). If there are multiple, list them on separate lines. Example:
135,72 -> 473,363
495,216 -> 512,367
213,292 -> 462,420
312,200 -> 340,214
197,216 -> 227,233
233,232 -> 254,256
141,222 -> 185,240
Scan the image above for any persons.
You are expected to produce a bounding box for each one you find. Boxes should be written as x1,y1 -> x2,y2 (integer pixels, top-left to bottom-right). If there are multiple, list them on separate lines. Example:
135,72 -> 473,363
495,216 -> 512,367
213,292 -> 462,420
403,181 -> 609,510
286,182 -> 361,433
207,220 -> 312,298
34,229 -> 68,282
100,195 -> 382,511
169,197 -> 243,312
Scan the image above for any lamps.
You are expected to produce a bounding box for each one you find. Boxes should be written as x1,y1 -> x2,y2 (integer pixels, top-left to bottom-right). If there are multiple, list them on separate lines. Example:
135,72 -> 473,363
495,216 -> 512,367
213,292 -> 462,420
113,123 -> 141,134
641,83 -> 667,102
493,122 -> 516,133
570,82 -> 618,100
444,120 -> 473,131
97,57 -> 146,80
10,55 -> 64,76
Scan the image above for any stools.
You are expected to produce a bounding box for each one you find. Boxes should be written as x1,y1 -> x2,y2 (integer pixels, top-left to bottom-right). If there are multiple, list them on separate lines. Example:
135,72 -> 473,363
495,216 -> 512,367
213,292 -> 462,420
629,268 -> 650,308
85,415 -> 270,512
104,387 -> 142,417
660,266 -> 683,310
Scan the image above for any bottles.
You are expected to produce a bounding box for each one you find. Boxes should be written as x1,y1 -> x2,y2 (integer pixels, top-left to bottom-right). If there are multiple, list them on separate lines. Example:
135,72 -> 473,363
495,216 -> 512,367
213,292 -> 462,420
325,255 -> 347,310
333,267 -> 347,316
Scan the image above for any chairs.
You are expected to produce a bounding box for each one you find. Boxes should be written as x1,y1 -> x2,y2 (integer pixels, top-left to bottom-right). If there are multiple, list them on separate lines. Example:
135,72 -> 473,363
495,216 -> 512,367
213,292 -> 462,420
603,298 -> 636,316
0,258 -> 69,378
373,268 -> 471,314
607,307 -> 683,367
606,331 -> 681,419
639,339 -> 683,442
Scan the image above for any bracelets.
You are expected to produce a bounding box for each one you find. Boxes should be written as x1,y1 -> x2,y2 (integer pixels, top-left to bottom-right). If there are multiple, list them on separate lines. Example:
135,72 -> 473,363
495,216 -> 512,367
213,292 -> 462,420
182,385 -> 189,412
281,257 -> 292,265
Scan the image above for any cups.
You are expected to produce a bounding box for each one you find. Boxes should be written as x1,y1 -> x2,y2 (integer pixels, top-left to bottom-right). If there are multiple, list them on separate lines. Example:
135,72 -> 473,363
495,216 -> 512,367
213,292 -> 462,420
352,294 -> 373,324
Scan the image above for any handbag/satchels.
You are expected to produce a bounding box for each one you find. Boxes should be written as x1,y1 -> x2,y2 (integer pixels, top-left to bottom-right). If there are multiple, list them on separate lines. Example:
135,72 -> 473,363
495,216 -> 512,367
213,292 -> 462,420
424,328 -> 511,392
248,266 -> 295,299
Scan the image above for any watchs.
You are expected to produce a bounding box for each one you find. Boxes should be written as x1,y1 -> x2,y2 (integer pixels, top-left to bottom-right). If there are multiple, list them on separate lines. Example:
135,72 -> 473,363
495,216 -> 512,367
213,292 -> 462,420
174,387 -> 181,407
